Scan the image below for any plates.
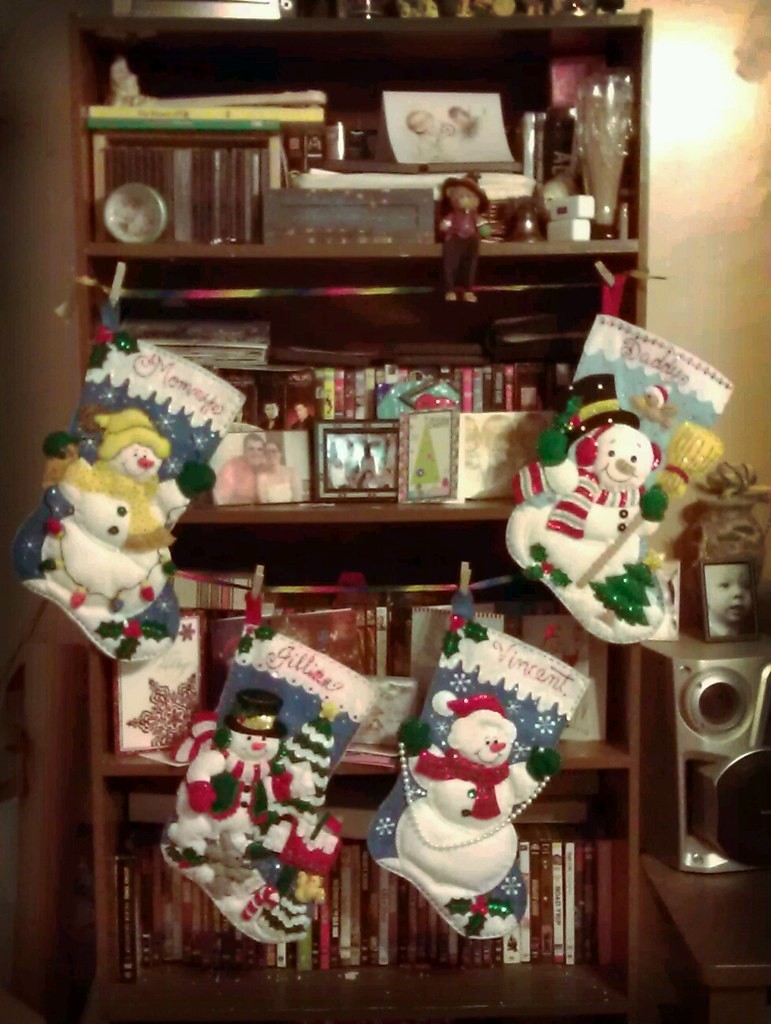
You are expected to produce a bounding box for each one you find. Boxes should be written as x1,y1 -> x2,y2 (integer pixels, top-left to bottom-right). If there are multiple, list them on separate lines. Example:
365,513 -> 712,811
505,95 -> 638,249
103,183 -> 167,244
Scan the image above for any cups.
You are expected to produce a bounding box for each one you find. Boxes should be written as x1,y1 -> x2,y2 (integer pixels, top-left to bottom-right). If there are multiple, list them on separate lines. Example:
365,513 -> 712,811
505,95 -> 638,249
337,0 -> 386,21
578,75 -> 633,239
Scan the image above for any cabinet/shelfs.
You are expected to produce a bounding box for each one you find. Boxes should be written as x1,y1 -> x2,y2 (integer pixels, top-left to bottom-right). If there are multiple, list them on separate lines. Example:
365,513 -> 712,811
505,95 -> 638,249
0,0 -> 652,1024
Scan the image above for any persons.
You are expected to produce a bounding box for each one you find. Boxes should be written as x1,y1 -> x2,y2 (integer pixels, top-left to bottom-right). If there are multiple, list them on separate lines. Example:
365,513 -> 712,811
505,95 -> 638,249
345,441 -> 358,483
447,104 -> 482,139
357,470 -> 375,488
407,109 -> 449,161
253,441 -> 301,504
361,445 -> 375,478
217,434 -> 264,505
438,168 -> 492,302
706,564 -> 754,637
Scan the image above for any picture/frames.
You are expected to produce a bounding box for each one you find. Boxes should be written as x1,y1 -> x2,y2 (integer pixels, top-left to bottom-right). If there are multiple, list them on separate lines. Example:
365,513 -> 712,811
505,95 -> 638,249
308,418 -> 399,504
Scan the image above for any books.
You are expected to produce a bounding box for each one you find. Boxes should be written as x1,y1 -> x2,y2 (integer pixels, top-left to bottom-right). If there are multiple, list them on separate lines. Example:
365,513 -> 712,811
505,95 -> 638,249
91,85 -> 609,973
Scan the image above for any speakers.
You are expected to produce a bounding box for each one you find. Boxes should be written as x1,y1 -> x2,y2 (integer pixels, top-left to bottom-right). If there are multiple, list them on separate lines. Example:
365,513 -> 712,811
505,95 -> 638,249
638,634 -> 771,872
